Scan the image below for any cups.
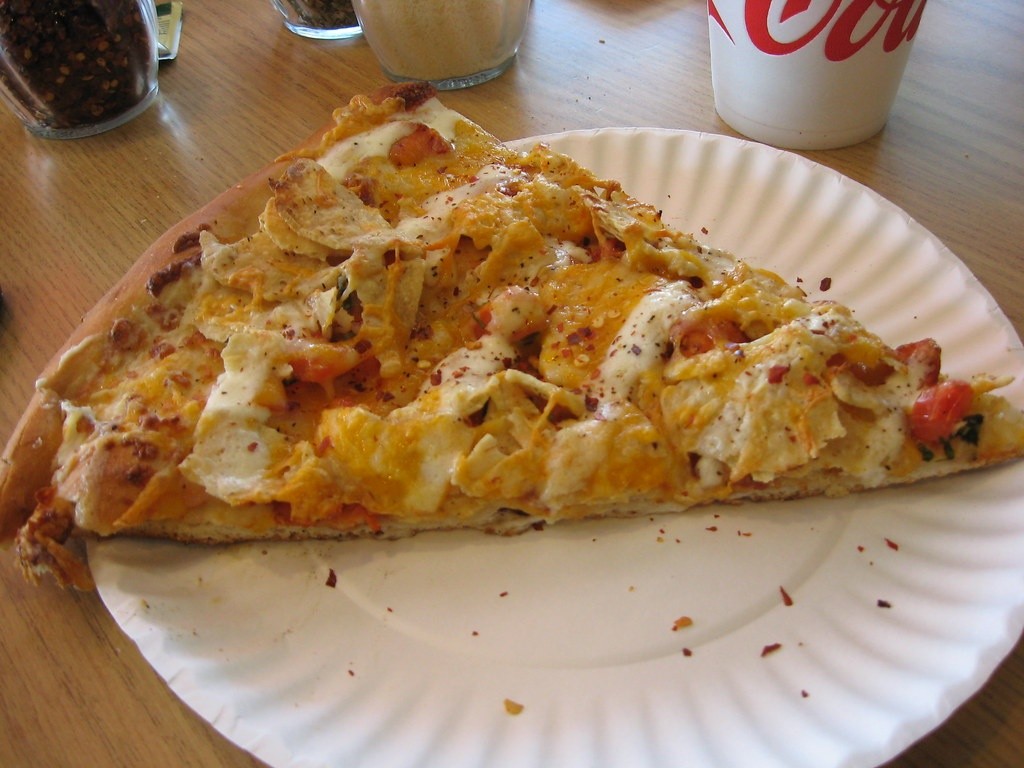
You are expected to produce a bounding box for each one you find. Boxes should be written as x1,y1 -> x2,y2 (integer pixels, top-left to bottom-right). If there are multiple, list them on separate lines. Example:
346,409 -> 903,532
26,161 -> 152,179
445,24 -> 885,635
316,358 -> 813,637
708,0 -> 926,150
271,0 -> 361,39
351,0 -> 534,91
0,0 -> 159,138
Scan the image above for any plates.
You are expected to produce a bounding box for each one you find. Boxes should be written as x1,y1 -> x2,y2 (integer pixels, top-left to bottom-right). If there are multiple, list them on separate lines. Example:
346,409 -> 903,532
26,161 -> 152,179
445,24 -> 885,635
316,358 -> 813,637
85,127 -> 1024,768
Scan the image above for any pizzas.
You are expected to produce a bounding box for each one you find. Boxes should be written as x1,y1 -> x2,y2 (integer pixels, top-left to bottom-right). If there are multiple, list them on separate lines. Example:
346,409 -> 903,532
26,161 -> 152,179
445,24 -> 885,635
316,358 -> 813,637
0,78 -> 1024,596
